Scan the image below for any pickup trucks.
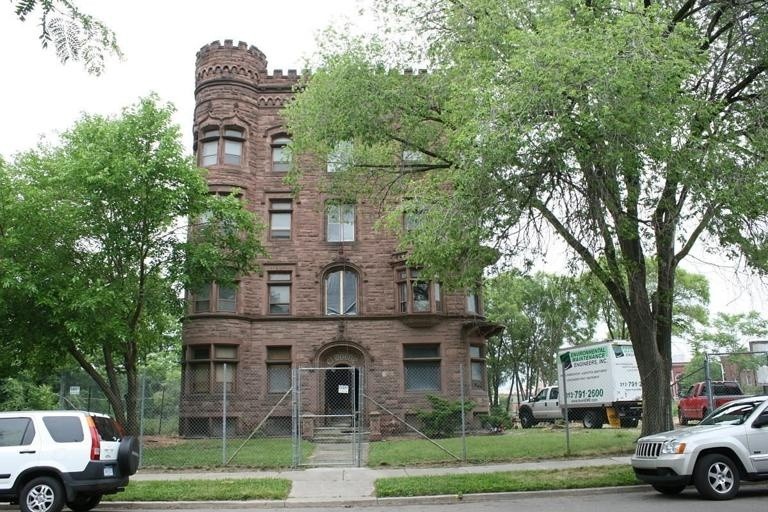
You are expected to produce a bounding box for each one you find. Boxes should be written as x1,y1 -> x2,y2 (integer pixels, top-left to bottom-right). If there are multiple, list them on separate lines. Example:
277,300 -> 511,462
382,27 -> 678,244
676,379 -> 755,426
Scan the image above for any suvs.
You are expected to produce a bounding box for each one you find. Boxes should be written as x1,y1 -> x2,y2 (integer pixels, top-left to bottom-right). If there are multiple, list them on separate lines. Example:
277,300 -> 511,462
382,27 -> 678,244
629,393 -> 768,500
0,409 -> 142,511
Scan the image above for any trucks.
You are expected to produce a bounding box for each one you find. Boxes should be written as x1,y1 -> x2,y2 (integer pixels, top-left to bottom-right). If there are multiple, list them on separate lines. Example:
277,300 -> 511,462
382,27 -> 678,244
515,337 -> 643,430
